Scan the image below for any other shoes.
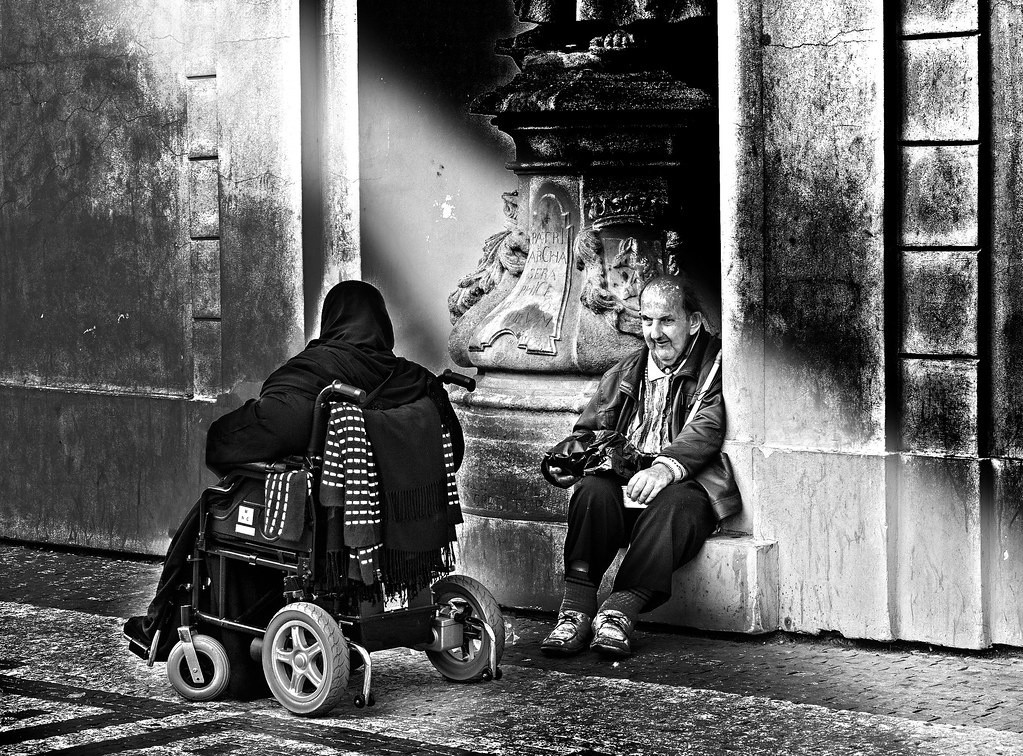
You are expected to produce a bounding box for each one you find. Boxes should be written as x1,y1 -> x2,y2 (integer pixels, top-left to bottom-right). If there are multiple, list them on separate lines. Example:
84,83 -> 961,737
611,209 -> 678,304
540,607 -> 591,656
590,605 -> 633,657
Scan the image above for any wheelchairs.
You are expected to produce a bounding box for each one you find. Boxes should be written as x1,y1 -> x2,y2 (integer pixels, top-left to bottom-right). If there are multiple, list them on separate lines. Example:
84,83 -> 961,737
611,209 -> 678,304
132,367 -> 505,715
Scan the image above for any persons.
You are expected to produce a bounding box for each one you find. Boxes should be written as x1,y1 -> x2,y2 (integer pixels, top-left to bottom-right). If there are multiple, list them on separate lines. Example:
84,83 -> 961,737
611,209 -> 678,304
541,274 -> 744,656
124,280 -> 464,656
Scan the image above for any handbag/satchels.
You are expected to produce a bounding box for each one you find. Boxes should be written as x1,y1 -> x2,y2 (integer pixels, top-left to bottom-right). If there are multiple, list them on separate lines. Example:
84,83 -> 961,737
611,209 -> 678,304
541,430 -> 642,489
